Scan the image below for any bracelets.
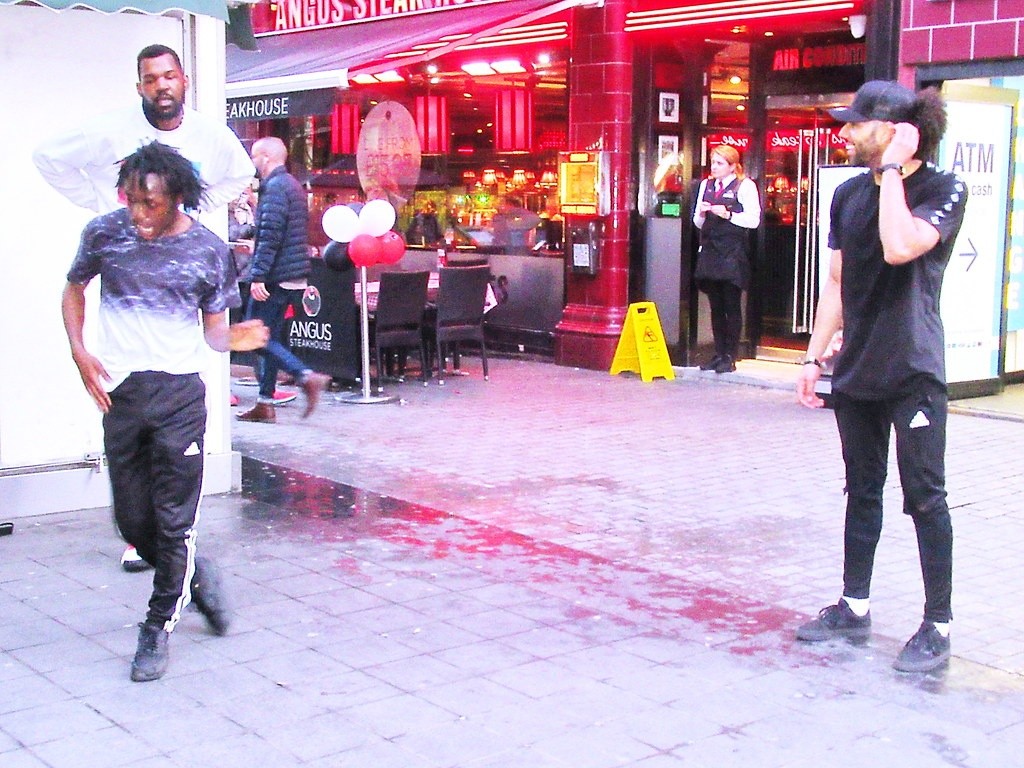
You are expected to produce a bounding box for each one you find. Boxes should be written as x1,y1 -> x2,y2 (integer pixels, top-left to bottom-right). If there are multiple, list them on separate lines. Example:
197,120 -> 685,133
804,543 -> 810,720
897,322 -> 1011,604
805,356 -> 821,367
728,211 -> 732,221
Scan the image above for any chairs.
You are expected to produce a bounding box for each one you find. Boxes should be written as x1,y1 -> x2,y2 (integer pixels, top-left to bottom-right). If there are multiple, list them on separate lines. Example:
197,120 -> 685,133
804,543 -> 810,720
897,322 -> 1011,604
352,259 -> 493,394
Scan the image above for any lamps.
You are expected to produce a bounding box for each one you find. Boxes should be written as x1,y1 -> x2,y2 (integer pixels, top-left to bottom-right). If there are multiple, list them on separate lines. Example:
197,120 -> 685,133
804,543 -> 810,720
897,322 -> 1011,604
330,76 -> 569,188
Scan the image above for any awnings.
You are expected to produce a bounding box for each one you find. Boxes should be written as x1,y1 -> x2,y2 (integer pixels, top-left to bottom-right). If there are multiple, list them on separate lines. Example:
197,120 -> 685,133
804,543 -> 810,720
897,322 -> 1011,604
225,0 -> 582,124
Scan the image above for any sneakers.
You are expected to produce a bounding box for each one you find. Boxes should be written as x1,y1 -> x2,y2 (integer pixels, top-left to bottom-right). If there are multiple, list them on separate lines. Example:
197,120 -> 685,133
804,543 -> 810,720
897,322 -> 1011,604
892,621 -> 951,672
121,544 -> 150,571
797,598 -> 872,642
132,625 -> 171,681
193,558 -> 232,634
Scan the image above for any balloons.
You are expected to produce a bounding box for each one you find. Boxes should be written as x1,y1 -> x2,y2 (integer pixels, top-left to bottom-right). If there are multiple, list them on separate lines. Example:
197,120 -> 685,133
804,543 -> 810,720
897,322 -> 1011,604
322,200 -> 406,272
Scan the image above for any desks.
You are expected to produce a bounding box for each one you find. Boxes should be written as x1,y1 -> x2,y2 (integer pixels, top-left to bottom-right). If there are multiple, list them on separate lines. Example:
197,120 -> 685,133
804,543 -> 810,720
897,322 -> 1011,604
352,271 -> 498,384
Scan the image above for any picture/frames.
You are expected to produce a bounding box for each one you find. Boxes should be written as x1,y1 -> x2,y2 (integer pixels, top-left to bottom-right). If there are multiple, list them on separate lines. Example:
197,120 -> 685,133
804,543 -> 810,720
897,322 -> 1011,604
659,92 -> 679,124
658,135 -> 679,164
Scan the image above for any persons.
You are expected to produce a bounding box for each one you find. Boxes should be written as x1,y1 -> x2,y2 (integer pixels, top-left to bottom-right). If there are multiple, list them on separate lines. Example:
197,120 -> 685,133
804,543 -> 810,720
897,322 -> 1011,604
405,199 -> 445,245
59,140 -> 271,682
230,135 -> 327,423
492,196 -> 540,246
800,77 -> 967,672
692,145 -> 761,374
32,43 -> 257,570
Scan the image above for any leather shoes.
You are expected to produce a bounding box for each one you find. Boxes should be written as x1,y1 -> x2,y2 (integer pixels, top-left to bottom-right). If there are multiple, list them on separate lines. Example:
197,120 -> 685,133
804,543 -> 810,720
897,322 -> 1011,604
234,402 -> 277,424
300,373 -> 333,422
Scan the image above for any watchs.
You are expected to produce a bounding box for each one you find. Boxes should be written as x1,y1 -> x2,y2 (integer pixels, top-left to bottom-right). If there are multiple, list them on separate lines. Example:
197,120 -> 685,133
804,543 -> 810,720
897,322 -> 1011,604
876,161 -> 905,175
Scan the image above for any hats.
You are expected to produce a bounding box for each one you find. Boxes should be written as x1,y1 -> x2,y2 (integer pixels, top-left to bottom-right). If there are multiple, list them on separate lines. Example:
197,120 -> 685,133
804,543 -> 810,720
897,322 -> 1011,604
828,79 -> 917,123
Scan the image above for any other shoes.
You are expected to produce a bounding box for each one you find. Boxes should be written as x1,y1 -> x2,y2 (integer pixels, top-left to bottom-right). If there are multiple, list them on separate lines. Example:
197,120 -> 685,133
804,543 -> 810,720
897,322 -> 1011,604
700,354 -> 726,370
715,356 -> 737,373
